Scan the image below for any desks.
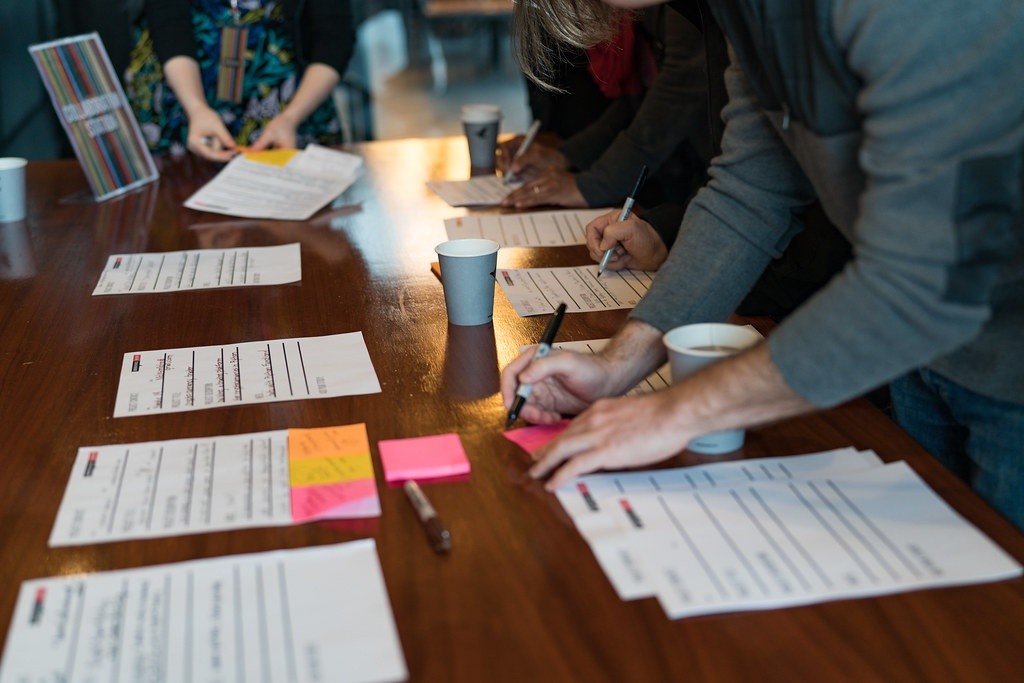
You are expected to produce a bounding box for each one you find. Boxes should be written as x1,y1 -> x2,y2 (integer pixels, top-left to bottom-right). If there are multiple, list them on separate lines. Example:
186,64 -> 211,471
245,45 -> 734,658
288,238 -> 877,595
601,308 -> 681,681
0,148 -> 1024,682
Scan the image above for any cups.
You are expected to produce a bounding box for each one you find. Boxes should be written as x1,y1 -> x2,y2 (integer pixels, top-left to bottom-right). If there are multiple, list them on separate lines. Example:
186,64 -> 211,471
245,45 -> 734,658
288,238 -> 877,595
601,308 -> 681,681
1,156 -> 30,224
434,238 -> 500,327
0,219 -> 38,281
460,104 -> 503,168
662,323 -> 760,455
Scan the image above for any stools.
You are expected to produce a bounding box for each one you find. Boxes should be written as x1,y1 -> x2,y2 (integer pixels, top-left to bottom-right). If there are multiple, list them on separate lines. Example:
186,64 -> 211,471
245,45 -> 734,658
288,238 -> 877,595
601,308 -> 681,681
419,1 -> 516,93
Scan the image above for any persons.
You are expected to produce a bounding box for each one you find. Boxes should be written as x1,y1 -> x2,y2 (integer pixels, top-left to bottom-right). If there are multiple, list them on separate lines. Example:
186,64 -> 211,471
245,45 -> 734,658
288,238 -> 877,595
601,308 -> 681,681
494,0 -> 1024,538
124,0 -> 357,164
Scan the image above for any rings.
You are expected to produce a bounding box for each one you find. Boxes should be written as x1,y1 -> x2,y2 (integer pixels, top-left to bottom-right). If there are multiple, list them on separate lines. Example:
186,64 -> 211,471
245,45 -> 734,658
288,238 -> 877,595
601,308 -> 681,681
496,148 -> 502,156
534,186 -> 539,194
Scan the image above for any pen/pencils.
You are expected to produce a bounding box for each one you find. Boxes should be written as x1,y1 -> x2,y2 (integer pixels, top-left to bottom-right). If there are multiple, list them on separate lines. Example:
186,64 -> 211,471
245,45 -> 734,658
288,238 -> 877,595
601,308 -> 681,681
199,138 -> 212,145
504,301 -> 569,430
403,482 -> 452,557
597,165 -> 647,279
503,119 -> 540,186
